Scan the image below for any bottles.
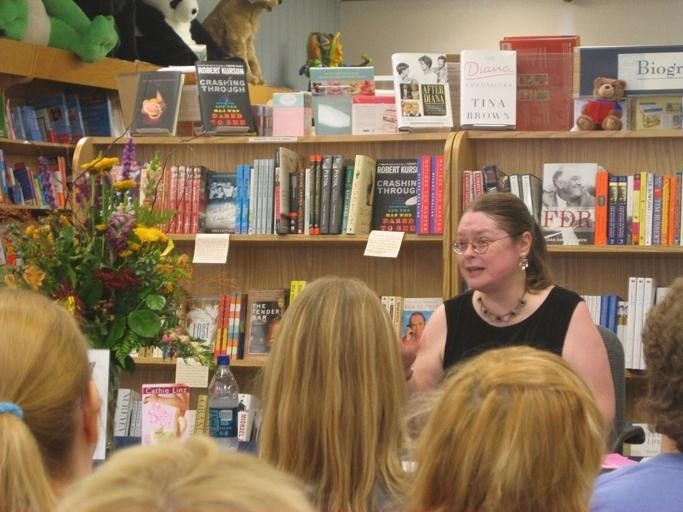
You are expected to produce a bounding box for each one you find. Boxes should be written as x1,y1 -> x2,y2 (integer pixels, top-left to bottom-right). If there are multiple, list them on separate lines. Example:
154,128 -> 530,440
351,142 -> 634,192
205,355 -> 239,456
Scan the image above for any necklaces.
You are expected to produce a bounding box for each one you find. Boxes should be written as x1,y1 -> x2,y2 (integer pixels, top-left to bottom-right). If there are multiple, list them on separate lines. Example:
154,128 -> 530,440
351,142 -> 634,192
475,285 -> 530,324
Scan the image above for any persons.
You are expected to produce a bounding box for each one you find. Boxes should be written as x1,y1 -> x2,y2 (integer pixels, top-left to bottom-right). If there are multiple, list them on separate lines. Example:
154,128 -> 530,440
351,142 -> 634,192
54,436 -> 318,512
402,313 -> 425,344
407,347 -> 605,511
253,277 -> 415,512
395,190 -> 616,439
588,276 -> 683,512
2,286 -> 104,512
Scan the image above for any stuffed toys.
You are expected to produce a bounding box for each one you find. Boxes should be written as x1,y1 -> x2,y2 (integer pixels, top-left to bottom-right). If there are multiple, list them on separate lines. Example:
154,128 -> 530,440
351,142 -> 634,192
0,0 -> 119,62
134,0 -> 225,66
575,77 -> 627,132
202,0 -> 283,85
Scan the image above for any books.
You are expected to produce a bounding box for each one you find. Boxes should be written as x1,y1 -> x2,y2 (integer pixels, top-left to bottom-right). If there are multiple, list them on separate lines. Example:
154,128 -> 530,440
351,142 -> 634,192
82,347 -> 266,472
127,281 -> 309,358
307,52 -> 575,132
579,276 -> 670,371
138,148 -> 444,235
131,60 -> 312,138
1,89 -> 126,145
462,162 -> 682,246
2,153 -> 74,209
379,294 -> 443,344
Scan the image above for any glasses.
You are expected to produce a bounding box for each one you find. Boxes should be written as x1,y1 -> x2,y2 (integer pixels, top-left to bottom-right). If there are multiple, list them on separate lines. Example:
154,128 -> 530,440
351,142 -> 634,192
451,234 -> 519,254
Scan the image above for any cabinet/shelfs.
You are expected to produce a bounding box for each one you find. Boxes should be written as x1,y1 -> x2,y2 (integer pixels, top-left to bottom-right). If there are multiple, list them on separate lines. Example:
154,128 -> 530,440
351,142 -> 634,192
0,38 -> 683,378
